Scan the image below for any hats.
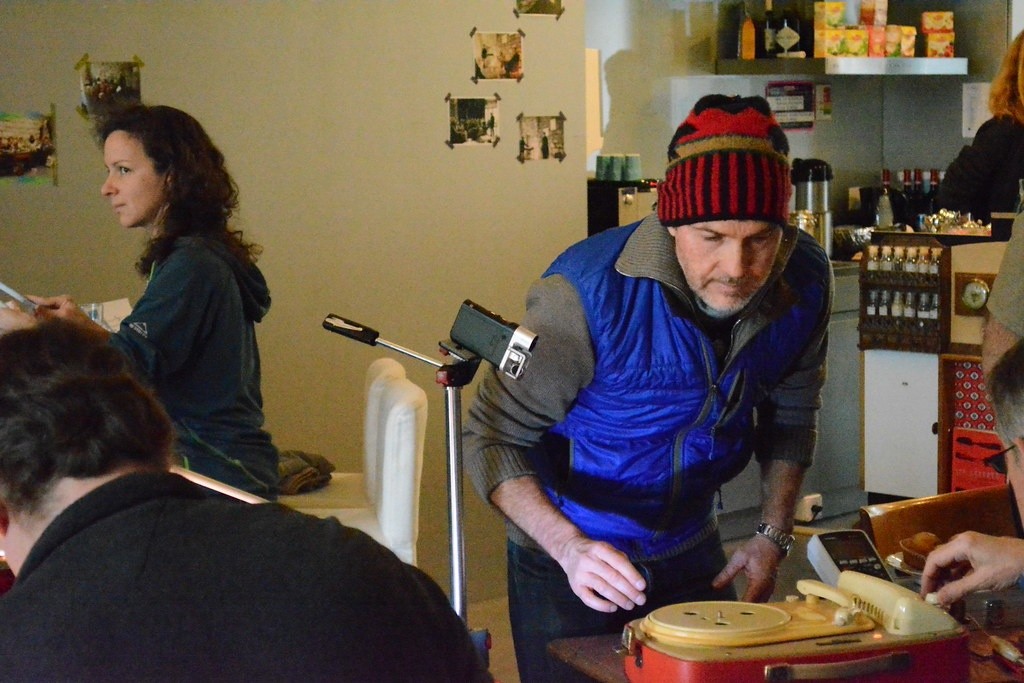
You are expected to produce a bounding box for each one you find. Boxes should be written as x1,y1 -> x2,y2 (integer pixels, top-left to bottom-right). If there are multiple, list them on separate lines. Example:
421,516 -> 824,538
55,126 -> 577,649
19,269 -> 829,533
655,93 -> 794,229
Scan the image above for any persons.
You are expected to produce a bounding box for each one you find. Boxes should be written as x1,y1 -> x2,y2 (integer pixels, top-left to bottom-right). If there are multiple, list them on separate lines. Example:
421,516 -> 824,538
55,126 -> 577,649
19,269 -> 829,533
84,68 -> 130,100
0,132 -> 56,167
0,318 -> 496,682
937,25 -> 1024,241
1,102 -> 282,503
980,210 -> 1024,387
919,337 -> 1023,609
462,92 -> 838,682
540,131 -> 549,159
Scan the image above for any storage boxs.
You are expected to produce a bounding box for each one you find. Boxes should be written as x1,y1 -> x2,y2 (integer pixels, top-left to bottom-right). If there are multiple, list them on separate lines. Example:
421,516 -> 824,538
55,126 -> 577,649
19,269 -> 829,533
813,0 -> 955,57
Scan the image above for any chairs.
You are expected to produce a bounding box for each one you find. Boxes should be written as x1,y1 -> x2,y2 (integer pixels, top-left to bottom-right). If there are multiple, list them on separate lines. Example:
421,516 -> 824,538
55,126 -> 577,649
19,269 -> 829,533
859,481 -> 1024,562
288,378 -> 428,566
274,357 -> 403,507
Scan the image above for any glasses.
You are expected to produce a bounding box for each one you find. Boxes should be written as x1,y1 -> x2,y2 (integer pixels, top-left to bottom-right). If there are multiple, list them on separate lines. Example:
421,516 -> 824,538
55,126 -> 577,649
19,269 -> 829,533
989,445 -> 1017,474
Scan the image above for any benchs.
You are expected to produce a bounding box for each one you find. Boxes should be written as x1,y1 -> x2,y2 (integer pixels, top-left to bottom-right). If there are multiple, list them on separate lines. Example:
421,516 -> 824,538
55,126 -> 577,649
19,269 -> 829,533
546,586 -> 1024,682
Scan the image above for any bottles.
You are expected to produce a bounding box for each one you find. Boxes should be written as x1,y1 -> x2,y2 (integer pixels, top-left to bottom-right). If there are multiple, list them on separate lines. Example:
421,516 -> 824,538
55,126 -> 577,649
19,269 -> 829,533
737,0 -> 757,61
776,12 -> 802,53
759,0 -> 775,57
865,244 -> 940,322
872,166 -> 939,229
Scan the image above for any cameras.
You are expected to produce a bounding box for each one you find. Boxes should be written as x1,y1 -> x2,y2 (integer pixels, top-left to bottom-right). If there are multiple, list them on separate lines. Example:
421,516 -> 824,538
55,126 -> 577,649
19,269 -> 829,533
451,298 -> 541,378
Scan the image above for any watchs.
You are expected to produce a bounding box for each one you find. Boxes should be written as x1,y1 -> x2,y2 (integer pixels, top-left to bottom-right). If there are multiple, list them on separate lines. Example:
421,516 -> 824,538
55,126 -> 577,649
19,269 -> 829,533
753,520 -> 795,558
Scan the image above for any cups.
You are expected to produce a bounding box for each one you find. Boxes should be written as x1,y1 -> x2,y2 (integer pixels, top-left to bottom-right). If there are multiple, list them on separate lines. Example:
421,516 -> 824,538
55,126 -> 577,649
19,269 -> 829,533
79,301 -> 104,326
596,152 -> 642,182
